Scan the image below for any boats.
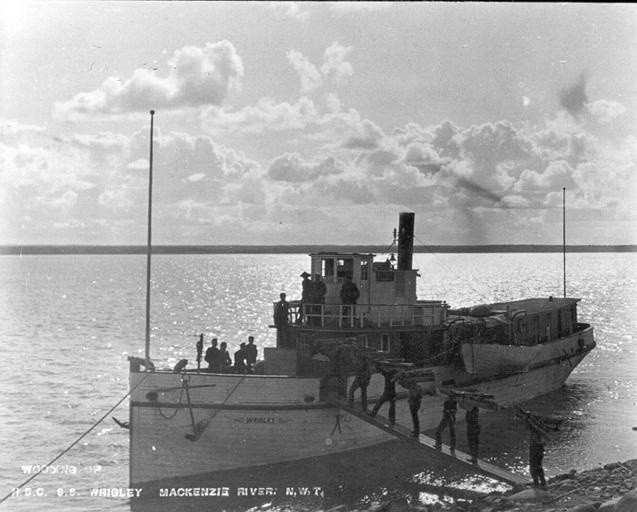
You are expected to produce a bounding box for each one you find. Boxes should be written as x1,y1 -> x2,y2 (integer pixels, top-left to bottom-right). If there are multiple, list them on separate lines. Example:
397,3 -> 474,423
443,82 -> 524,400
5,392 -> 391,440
127,108 -> 597,488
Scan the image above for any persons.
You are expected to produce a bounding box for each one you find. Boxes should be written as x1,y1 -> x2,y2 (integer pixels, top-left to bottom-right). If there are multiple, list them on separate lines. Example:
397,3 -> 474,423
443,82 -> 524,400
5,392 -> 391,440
310,272 -> 327,325
234,342 -> 247,368
464,408 -> 480,463
219,341 -> 232,366
246,335 -> 257,369
527,427 -> 545,486
204,338 -> 219,371
296,271 -> 312,323
340,276 -> 360,327
434,394 -> 457,453
317,345 -> 422,438
274,293 -> 290,349
